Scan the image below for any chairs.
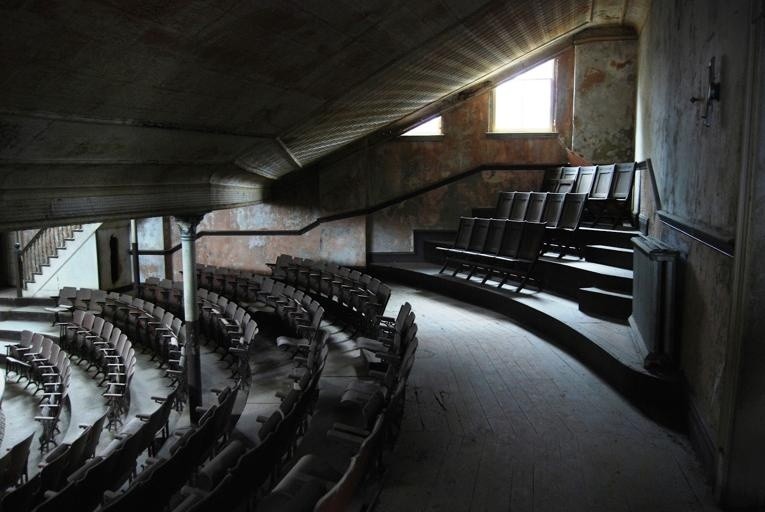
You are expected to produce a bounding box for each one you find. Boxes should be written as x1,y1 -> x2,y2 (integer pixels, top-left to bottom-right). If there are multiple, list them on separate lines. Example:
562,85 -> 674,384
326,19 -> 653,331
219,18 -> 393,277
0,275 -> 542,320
540,162 -> 636,227
436,215 -> 546,296
494,192 -> 588,260
